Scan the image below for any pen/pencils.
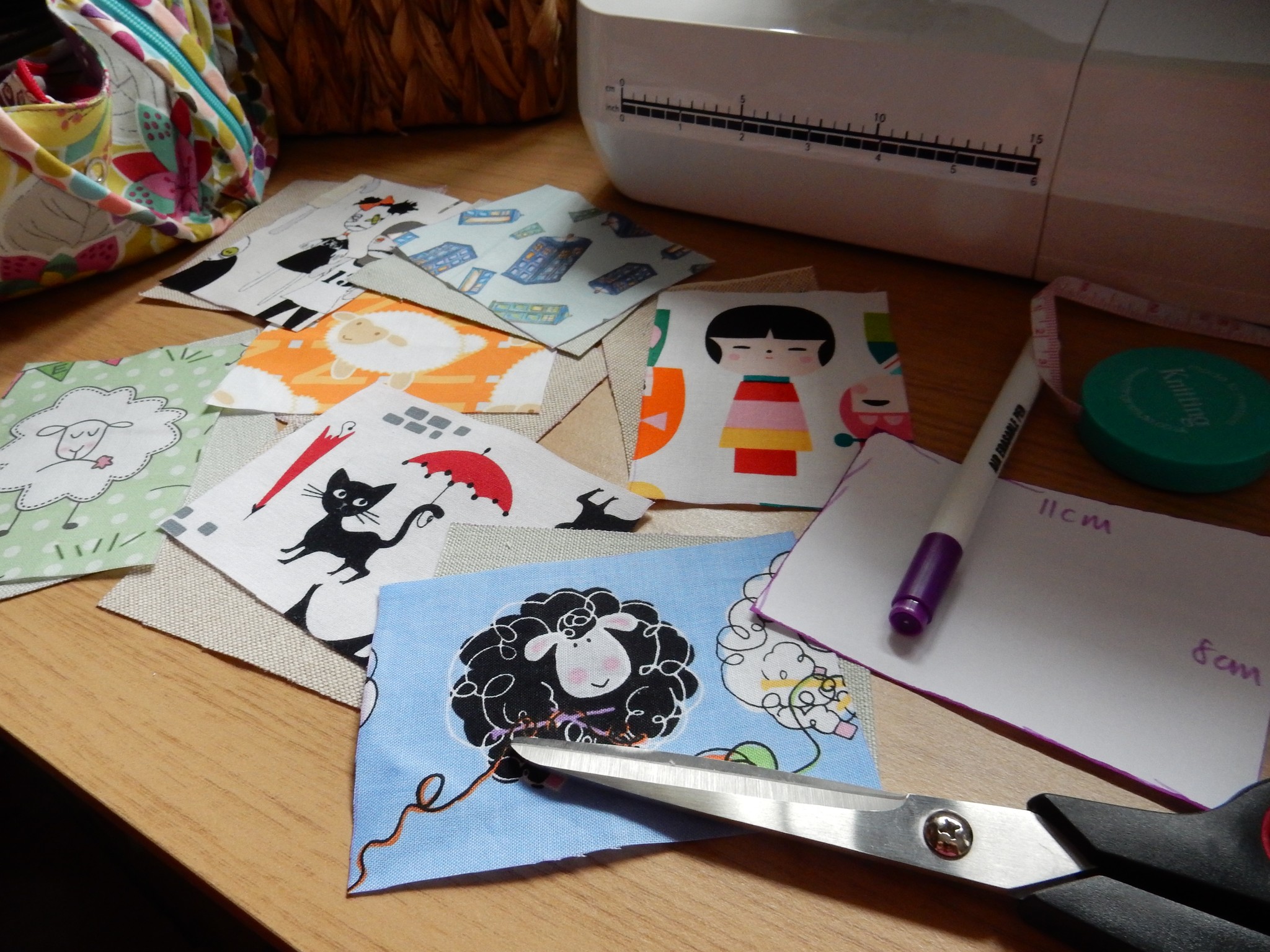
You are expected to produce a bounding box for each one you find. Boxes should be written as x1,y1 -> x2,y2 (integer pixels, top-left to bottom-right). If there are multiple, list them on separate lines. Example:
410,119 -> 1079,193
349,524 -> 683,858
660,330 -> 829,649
889,335 -> 1064,634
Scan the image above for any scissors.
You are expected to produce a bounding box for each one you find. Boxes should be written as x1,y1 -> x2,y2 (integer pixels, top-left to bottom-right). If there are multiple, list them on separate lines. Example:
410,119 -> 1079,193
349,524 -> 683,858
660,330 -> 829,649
508,736 -> 1270,952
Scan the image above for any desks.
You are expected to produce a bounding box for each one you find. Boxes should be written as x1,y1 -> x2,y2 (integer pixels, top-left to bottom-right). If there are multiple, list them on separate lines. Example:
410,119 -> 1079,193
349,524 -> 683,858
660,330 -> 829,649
0,97 -> 1270,948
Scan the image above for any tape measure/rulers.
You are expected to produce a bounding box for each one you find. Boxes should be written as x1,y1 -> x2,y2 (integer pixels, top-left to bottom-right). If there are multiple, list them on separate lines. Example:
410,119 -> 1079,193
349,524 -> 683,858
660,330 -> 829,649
1034,277 -> 1270,493
